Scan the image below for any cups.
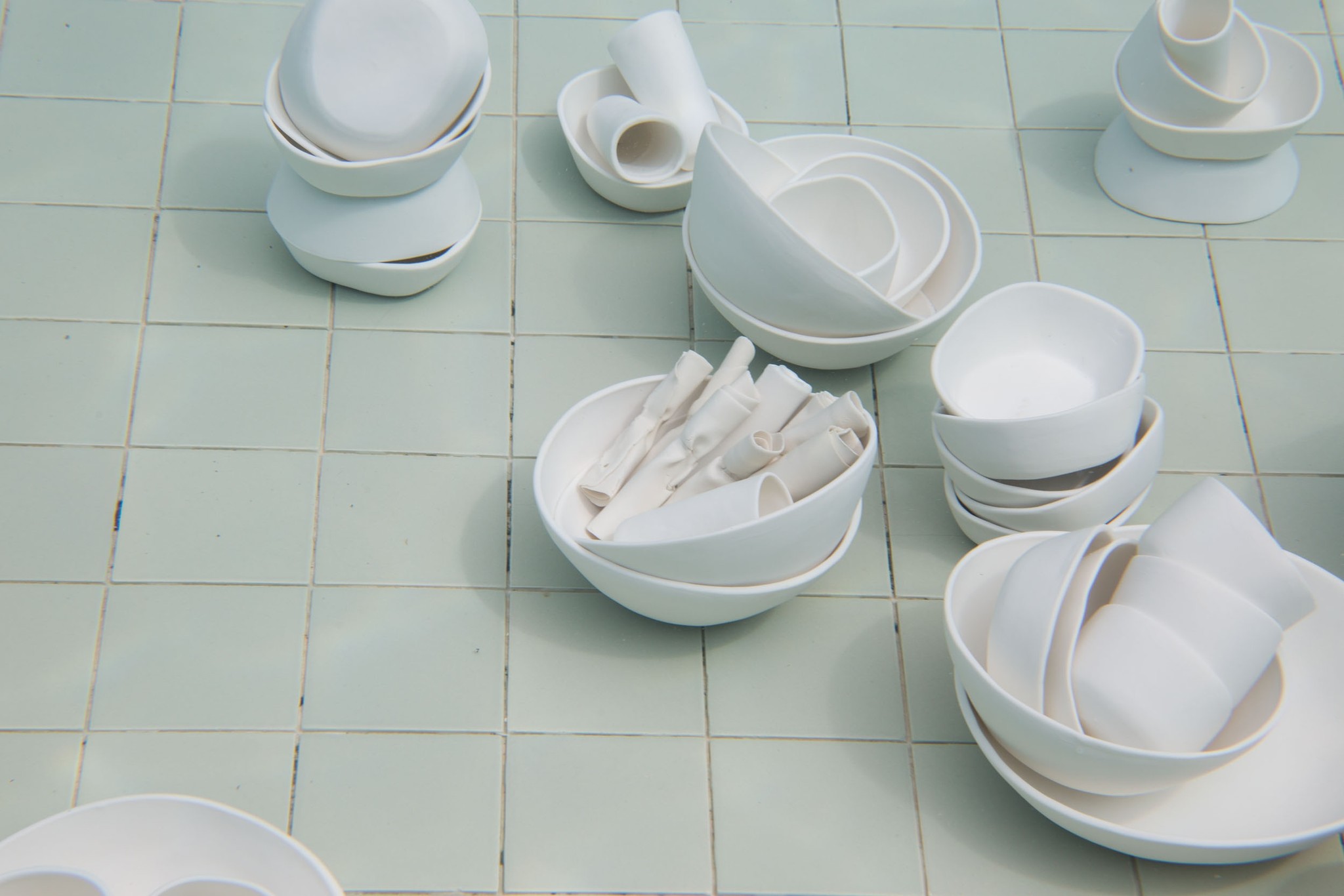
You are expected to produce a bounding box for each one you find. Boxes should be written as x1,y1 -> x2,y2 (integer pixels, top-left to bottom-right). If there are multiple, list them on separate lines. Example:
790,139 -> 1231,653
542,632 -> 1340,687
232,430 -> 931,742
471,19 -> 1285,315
152,877 -> 276,896
0,869 -> 107,896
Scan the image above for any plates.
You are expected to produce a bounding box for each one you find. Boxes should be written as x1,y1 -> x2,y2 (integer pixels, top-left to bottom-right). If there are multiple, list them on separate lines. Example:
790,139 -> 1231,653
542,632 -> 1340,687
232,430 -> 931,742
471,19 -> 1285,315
0,793 -> 343,896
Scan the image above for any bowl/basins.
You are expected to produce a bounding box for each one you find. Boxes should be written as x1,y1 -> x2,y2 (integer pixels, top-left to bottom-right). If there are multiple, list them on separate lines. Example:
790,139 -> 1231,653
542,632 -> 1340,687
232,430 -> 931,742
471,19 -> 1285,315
530,1 -> 1344,865
266,0 -> 495,300
